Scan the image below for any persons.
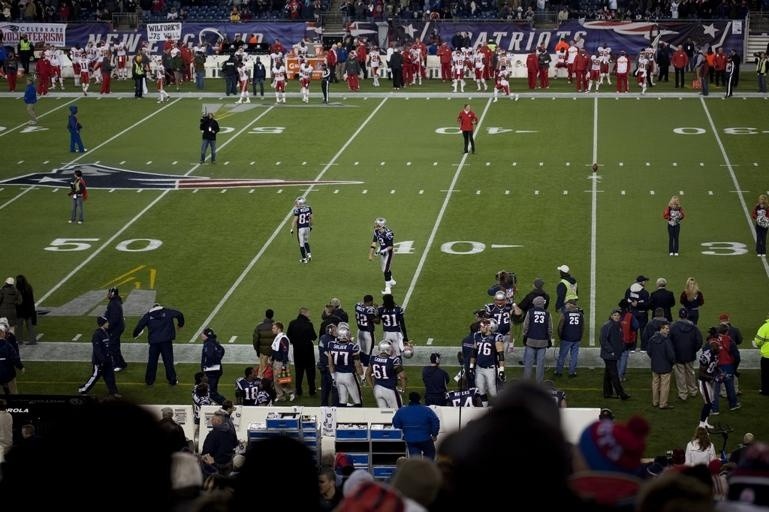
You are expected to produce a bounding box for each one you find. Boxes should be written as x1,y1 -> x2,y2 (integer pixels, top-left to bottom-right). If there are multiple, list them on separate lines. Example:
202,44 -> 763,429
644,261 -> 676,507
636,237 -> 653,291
0,401 -> 14,463
750,193 -> 769,257
662,193 -> 686,256
75,315 -> 125,399
65,102 -> 88,152
21,75 -> 38,126
1,272 -> 39,395
455,104 -> 479,155
367,215 -> 398,294
169,266 -> 769,511
67,169 -> 87,224
1,1 -> 768,107
159,406 -> 187,445
132,303 -> 184,387
102,287 -> 128,373
288,194 -> 314,265
0,396 -> 177,511
198,113 -> 220,165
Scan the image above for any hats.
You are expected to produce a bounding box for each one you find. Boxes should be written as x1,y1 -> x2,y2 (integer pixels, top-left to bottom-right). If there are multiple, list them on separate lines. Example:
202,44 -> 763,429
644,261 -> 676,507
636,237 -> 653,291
0,317 -> 10,335
203,327 -> 216,338
725,443 -> 769,507
95,316 -> 108,328
678,307 -> 688,320
576,412 -> 654,475
718,312 -> 729,322
4,276 -> 16,286
162,407 -> 175,418
635,275 -> 650,283
671,447 -> 686,464
531,263 -> 576,308
609,298 -> 630,316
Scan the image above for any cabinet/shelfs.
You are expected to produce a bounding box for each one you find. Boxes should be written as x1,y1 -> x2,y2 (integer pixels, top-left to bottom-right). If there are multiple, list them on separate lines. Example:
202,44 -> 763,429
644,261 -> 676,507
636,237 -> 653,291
240,409 -> 323,478
331,418 -> 412,485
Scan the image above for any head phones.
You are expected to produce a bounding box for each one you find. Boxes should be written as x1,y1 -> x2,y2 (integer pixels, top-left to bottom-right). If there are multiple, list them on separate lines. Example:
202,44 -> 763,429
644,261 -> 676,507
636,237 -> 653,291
434,353 -> 441,364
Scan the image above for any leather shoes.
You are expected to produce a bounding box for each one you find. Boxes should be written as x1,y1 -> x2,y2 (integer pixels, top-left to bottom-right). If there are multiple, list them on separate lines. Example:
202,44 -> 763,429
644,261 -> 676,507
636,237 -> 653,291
603,392 -> 632,401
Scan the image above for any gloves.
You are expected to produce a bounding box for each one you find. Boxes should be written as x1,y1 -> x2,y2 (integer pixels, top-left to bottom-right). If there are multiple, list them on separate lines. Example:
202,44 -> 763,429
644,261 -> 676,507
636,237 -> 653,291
496,366 -> 507,383
468,368 -> 476,382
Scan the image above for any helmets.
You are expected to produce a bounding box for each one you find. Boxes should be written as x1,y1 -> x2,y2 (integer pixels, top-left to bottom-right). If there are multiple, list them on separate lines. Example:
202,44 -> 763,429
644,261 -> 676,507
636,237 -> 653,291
476,272 -> 509,336
329,298 -> 341,310
335,322 -> 353,341
374,217 -> 387,230
293,196 -> 307,208
376,339 -> 414,360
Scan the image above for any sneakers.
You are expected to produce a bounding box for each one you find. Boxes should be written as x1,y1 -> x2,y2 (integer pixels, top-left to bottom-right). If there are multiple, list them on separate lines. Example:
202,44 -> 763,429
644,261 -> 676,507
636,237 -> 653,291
651,388 -> 769,416
380,279 -> 397,296
16,339 -> 39,345
35,70 -> 205,106
227,91 -> 330,106
114,363 -> 128,372
492,97 -> 498,103
756,253 -> 767,257
298,253 -> 313,264
329,72 -> 549,93
553,75 -> 558,80
71,148 -> 88,154
669,252 -> 679,256
68,219 -> 84,224
274,391 -> 319,403
697,418 -> 715,438
513,94 -> 520,101
619,375 -> 627,382
199,160 -> 217,165
553,371 -> 578,377
566,76 -> 736,99
